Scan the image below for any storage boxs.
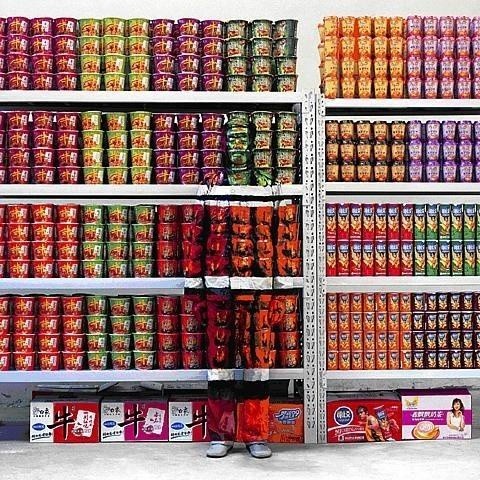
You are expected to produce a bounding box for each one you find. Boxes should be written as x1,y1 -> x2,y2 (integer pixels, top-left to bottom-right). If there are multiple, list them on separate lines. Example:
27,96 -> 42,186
168,395 -> 236,442
396,388 -> 473,441
101,397 -> 169,442
29,395 -> 100,443
237,397 -> 304,444
327,392 -> 402,442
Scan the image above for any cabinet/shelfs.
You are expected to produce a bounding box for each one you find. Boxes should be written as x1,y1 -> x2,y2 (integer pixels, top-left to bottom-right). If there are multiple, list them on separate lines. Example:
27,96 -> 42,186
315,93 -> 480,442
0,89 -> 316,447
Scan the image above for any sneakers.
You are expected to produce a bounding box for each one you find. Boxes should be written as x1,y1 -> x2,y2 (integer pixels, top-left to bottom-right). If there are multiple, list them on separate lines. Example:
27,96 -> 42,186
206,441 -> 233,457
245,441 -> 272,458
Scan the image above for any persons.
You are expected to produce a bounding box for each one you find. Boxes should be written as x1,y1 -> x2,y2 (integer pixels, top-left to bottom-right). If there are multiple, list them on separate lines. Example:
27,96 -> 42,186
183,383 -> 296,459
356,406 -> 399,442
447,398 -> 466,439
